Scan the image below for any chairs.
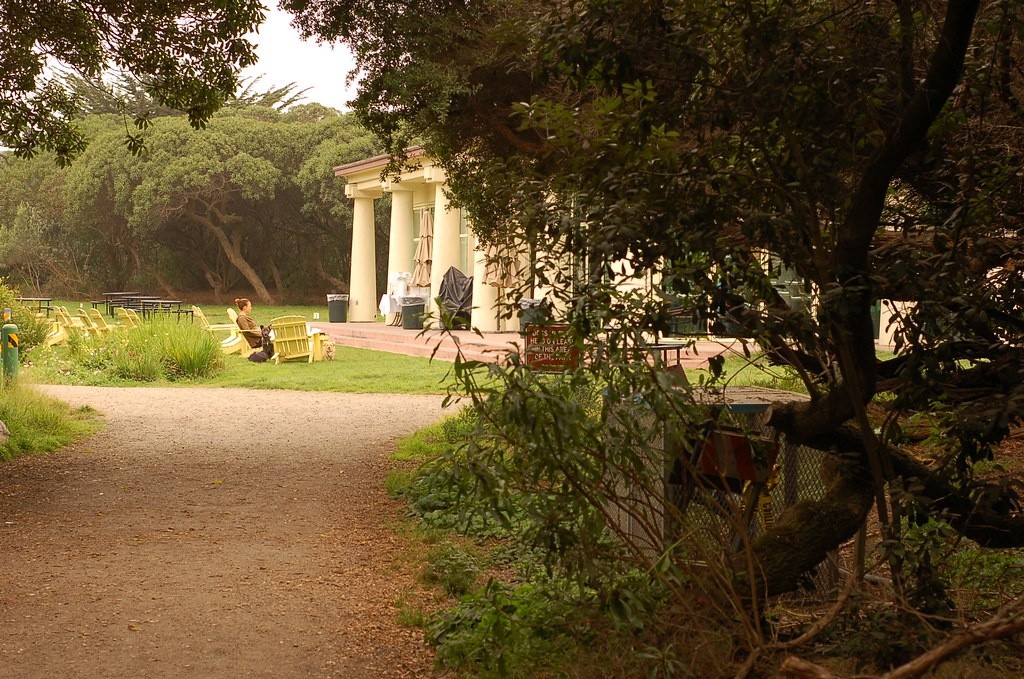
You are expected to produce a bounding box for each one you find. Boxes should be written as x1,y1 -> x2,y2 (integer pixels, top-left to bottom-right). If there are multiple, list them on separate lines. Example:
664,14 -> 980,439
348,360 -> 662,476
30,306 -> 147,349
192,304 -> 330,364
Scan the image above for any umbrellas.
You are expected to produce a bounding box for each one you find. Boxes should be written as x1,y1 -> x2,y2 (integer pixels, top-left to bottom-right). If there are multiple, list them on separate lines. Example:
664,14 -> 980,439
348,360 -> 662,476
409,209 -> 435,305
482,199 -> 522,330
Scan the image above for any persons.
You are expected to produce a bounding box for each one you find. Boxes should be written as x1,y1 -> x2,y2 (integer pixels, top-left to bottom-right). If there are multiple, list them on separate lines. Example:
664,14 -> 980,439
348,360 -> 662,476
234,297 -> 263,348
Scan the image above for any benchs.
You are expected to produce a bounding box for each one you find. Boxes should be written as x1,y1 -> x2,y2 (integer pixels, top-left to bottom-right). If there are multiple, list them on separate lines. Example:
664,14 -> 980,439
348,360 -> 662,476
27,306 -> 54,310
91,300 -> 195,324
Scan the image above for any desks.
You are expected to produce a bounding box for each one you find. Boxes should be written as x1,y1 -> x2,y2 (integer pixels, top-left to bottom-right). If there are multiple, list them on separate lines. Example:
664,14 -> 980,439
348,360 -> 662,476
141,300 -> 183,322
103,292 -> 140,315
387,296 -> 422,327
122,296 -> 161,309
14,298 -> 52,318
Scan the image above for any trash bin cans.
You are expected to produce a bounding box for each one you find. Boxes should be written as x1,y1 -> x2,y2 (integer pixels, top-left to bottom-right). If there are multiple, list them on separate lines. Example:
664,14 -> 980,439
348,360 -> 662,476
327,294 -> 349,322
517,298 -> 548,338
401,296 -> 426,329
